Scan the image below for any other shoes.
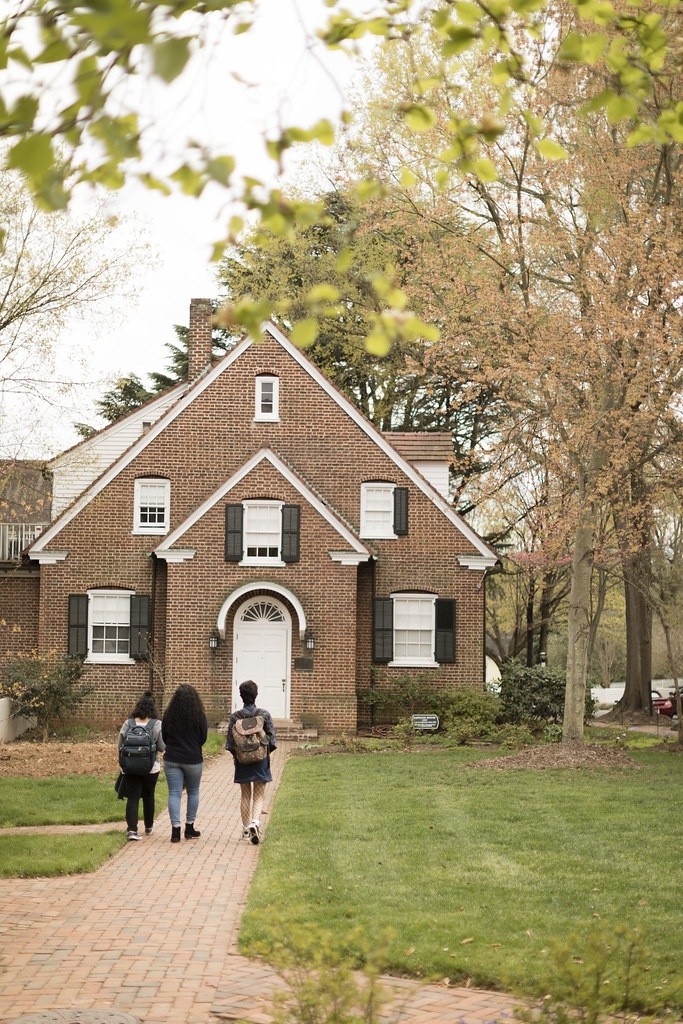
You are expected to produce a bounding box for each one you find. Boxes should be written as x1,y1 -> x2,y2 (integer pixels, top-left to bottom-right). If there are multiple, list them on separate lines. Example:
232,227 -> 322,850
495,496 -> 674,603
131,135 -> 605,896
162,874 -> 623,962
249,824 -> 260,845
145,828 -> 153,835
243,830 -> 250,841
127,832 -> 142,841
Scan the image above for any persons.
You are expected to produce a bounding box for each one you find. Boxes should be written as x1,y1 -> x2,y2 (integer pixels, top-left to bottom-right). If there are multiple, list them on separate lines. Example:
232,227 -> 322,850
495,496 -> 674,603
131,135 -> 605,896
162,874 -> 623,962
117,691 -> 166,841
225,680 -> 278,844
162,684 -> 207,843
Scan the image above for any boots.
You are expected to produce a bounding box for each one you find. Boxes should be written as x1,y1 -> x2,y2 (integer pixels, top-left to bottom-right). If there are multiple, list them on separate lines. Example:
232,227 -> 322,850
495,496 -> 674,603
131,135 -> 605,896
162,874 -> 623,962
184,822 -> 200,840
171,825 -> 181,843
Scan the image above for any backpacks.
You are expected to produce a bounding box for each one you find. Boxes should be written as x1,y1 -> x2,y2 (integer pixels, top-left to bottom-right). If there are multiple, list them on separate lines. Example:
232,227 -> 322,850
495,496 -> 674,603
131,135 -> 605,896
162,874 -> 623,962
120,718 -> 156,775
233,708 -> 269,765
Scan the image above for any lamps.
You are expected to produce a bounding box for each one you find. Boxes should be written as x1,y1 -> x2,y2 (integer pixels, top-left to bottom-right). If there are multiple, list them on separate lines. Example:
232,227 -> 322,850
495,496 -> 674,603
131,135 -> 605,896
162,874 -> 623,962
306,630 -> 315,649
209,631 -> 218,652
